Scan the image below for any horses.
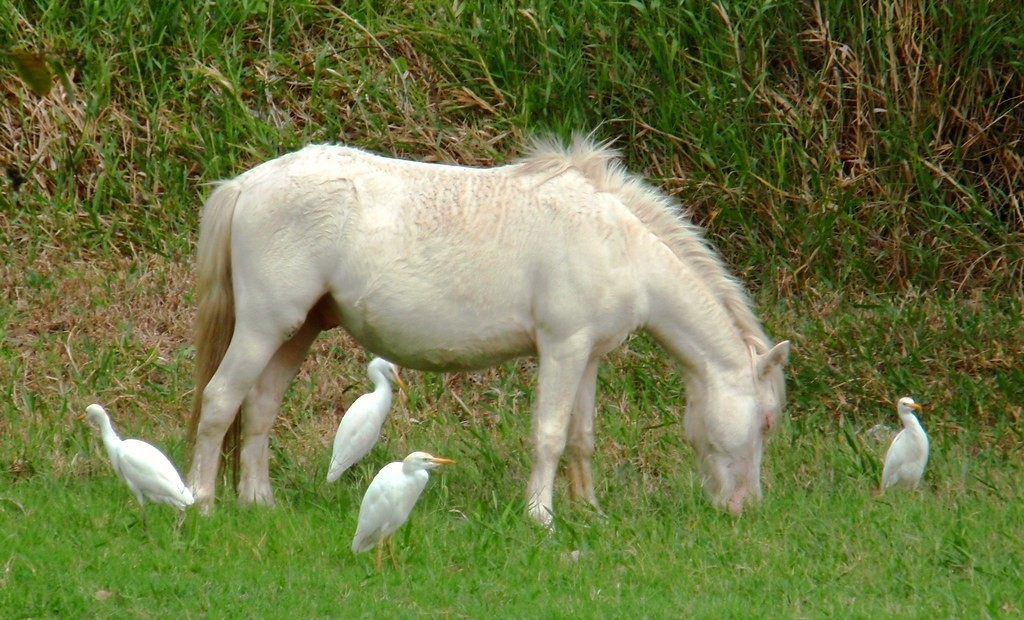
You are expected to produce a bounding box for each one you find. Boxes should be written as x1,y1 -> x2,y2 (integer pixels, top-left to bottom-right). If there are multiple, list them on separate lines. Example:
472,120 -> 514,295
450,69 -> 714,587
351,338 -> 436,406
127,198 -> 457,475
184,144 -> 789,535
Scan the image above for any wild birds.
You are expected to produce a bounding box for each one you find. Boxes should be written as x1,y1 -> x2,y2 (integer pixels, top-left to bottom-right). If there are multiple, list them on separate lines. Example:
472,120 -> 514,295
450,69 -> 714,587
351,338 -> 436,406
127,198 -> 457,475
350,450 -> 458,570
73,403 -> 195,538
880,397 -> 930,493
326,356 -> 407,496
